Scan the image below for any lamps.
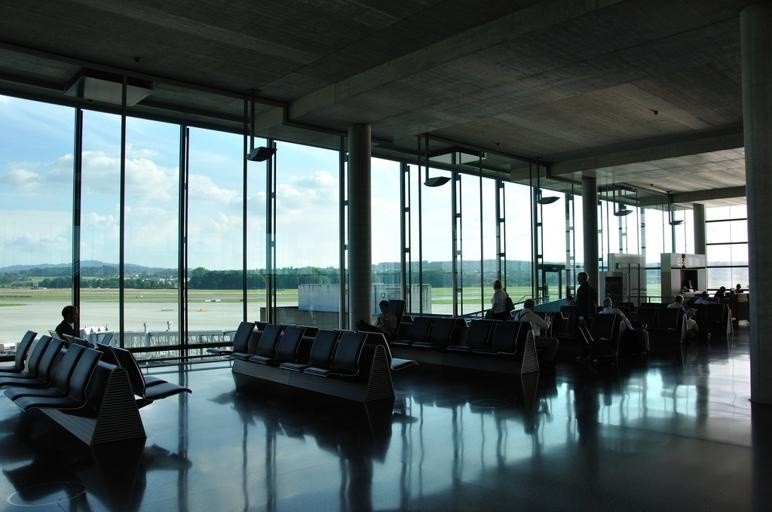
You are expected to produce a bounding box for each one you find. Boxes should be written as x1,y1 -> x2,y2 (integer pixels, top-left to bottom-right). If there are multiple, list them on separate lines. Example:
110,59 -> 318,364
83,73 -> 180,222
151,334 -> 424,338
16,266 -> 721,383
245,101 -> 277,161
424,134 -> 451,186
666,191 -> 683,225
613,184 -> 633,216
537,164 -> 560,204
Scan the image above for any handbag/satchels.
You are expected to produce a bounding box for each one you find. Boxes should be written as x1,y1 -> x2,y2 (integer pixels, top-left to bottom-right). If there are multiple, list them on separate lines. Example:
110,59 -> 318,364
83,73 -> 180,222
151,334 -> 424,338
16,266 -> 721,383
506,297 -> 515,312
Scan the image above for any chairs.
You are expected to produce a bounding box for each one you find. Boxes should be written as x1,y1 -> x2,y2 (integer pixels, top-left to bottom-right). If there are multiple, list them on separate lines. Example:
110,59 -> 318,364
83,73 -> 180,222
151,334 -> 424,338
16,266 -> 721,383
208,322 -> 419,414
0,331 -> 191,461
387,302 -> 728,377
263,418 -> 339,456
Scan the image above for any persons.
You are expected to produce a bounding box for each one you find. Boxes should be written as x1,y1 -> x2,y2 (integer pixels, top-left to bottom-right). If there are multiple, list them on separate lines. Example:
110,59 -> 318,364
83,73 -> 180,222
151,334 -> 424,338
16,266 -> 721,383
600,298 -> 632,329
374,300 -> 398,348
668,294 -> 688,313
576,273 -> 598,360
695,294 -> 712,305
56,305 -> 77,340
515,298 -> 552,337
489,280 -> 512,320
736,284 -> 744,292
716,286 -> 727,299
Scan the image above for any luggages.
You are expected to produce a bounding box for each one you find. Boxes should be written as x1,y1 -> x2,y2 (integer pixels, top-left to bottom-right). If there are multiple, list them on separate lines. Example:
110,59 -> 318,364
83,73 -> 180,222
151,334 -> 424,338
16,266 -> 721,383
577,322 -> 617,377
621,327 -> 650,367
